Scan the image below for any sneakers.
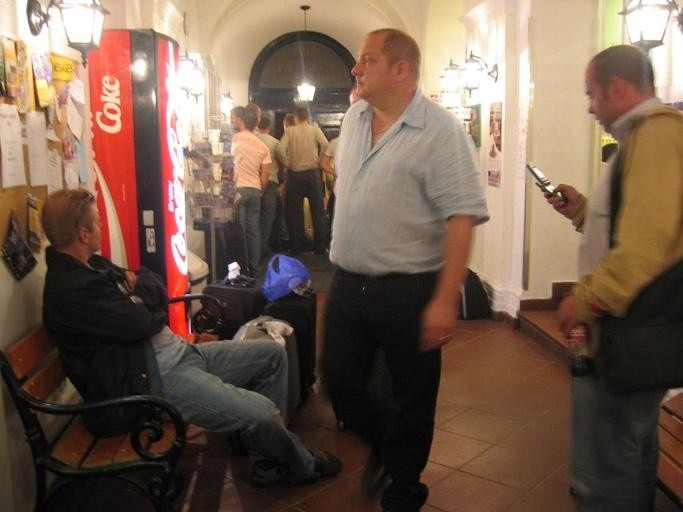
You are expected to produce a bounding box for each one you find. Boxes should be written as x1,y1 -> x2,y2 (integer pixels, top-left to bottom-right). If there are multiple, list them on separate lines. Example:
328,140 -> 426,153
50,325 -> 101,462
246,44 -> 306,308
303,444 -> 343,477
251,463 -> 323,487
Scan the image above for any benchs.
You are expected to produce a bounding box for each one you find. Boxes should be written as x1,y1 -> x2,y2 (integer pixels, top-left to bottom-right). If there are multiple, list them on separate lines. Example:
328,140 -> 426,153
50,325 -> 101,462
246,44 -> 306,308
0,293 -> 228,512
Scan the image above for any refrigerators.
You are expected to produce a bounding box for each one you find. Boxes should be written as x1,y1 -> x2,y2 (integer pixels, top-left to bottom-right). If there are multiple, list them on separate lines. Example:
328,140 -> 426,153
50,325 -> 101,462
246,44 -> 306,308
84,27 -> 194,342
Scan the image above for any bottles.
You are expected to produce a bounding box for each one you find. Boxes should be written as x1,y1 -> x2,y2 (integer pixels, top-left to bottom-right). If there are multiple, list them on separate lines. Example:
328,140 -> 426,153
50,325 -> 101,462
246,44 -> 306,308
561,290 -> 596,377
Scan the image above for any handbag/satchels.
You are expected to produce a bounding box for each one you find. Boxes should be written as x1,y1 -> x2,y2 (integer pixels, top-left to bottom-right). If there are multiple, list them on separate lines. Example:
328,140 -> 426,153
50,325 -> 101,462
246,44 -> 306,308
589,261 -> 683,395
458,267 -> 492,319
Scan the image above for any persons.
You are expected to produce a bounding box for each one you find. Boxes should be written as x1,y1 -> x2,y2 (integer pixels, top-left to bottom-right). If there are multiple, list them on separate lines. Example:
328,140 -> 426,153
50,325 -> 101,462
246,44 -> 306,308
544,46 -> 683,511
40,187 -> 342,487
228,87 -> 358,275
320,28 -> 489,511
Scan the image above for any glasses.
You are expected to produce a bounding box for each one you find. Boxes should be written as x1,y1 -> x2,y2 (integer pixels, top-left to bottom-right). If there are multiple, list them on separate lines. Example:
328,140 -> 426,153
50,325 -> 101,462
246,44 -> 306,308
78,186 -> 95,208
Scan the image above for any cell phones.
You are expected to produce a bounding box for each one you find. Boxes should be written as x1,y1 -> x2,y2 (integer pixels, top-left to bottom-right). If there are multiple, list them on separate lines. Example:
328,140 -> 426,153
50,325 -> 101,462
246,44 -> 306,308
527,162 -> 568,204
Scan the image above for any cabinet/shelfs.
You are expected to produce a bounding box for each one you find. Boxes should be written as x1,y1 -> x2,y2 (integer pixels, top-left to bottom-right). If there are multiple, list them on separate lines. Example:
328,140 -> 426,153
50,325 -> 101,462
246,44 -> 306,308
183,137 -> 249,285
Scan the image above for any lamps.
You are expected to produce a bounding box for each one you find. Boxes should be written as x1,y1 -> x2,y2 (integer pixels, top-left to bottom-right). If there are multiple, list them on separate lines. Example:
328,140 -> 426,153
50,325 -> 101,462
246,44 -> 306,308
26,1 -> 113,69
618,0 -> 683,56
442,58 -> 462,94
180,48 -> 205,103
297,80 -> 315,102
220,91 -> 234,115
463,50 -> 498,98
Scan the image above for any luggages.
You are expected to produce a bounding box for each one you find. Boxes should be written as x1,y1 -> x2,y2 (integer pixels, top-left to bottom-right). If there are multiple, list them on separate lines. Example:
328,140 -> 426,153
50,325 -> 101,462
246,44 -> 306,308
262,292 -> 317,399
202,275 -> 262,339
